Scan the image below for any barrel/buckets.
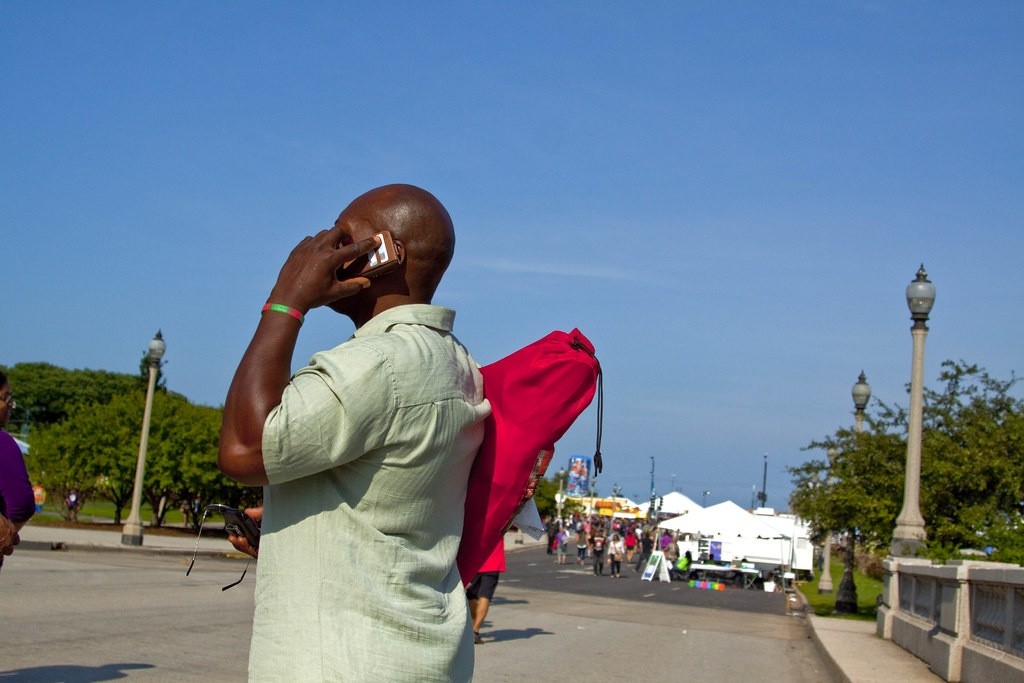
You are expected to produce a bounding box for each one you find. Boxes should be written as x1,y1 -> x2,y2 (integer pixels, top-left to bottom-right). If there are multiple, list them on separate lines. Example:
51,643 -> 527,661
764,582 -> 776,592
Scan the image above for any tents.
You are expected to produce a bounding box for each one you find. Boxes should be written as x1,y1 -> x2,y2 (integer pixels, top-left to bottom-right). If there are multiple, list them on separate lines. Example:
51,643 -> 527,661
656,499 -> 793,591
628,491 -> 704,517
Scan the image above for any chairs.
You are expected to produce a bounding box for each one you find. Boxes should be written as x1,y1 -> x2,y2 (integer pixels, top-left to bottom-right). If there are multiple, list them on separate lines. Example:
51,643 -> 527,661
669,561 -> 699,582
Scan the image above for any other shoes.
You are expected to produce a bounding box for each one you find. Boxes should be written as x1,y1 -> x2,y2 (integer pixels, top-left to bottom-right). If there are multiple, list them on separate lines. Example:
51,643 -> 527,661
472,631 -> 480,643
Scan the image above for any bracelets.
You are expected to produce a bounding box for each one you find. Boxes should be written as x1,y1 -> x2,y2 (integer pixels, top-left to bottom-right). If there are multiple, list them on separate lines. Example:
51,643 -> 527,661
262,301 -> 307,325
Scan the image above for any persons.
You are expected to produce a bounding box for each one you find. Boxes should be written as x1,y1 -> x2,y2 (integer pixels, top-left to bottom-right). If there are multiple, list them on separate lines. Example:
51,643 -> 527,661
180,496 -> 201,528
742,559 -> 765,584
65,489 -> 79,523
216,183 -> 506,683
689,554 -> 715,579
733,561 -> 743,582
540,506 -> 672,585
0,371 -> 36,567
670,551 -> 691,574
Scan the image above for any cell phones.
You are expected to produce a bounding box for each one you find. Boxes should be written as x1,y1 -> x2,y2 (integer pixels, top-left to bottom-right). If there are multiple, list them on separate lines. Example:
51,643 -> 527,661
336,231 -> 401,282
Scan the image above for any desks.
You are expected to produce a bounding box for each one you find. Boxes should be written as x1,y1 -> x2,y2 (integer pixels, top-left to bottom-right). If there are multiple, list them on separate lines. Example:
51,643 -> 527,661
691,564 -> 759,590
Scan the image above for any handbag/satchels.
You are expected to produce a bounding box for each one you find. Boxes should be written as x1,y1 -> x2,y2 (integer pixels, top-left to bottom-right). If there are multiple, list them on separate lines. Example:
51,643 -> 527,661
614,553 -> 623,562
455,327 -> 600,589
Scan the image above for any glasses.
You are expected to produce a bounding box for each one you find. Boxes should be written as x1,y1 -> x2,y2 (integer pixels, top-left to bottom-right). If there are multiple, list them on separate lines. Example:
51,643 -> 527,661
0,392 -> 12,403
186,504 -> 260,590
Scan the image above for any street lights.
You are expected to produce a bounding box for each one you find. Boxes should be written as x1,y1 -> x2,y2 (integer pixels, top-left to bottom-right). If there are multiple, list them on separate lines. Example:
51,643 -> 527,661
834,370 -> 872,614
891,261 -> 937,556
120,329 -> 167,547
700,490 -> 711,508
556,464 -> 565,517
590,476 -> 596,525
819,446 -> 838,594
